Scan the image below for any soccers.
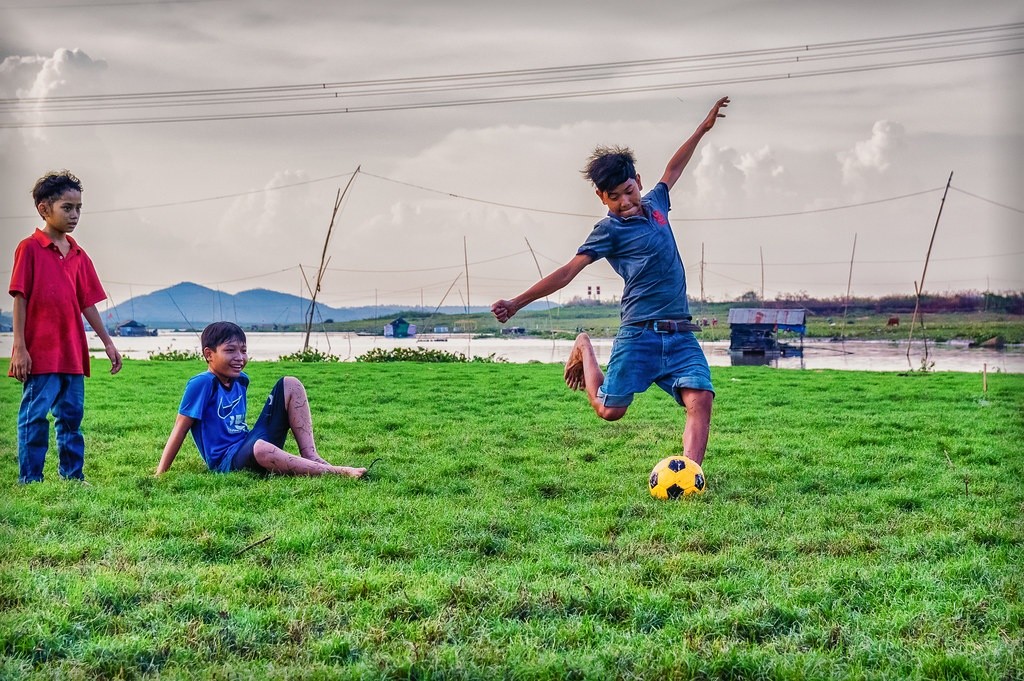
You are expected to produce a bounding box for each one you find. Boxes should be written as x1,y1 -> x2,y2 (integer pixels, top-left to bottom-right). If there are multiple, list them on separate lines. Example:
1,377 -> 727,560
648,455 -> 706,500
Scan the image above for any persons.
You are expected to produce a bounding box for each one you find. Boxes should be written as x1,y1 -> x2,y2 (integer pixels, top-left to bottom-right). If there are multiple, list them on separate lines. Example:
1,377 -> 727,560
490,97 -> 732,467
156,324 -> 366,478
8,170 -> 123,484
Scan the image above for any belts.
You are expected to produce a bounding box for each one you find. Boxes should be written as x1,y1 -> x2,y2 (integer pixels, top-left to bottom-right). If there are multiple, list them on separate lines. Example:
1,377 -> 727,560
631,320 -> 702,333
